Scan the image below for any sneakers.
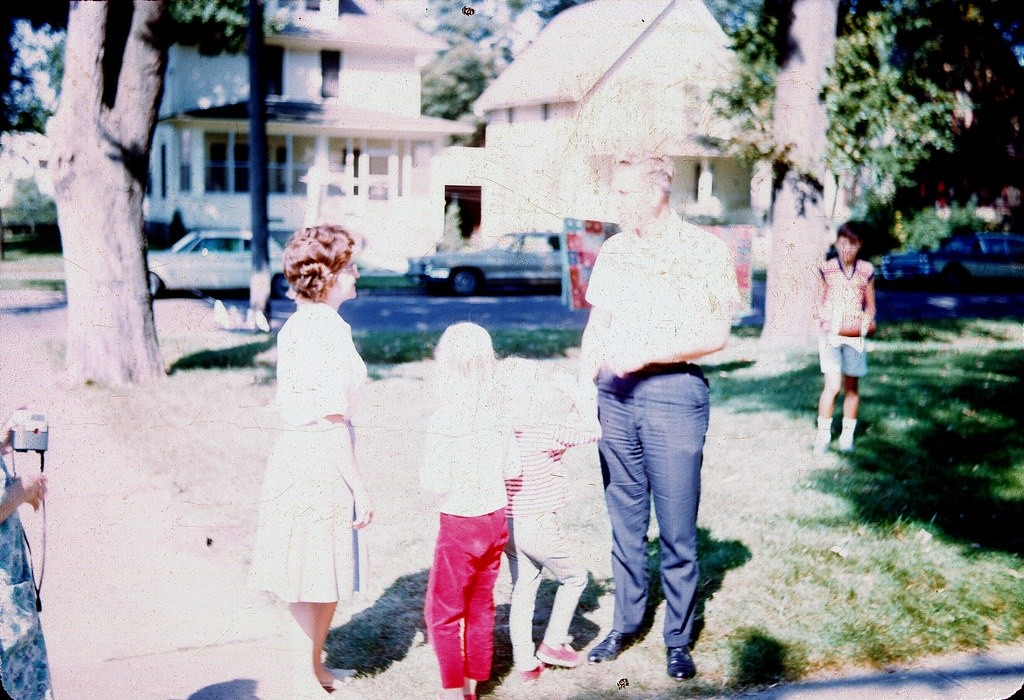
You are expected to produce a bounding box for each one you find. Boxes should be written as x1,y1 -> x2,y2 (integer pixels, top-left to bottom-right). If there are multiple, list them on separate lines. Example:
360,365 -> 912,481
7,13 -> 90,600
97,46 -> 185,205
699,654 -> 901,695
813,432 -> 833,455
521,662 -> 555,686
534,640 -> 583,669
838,434 -> 854,451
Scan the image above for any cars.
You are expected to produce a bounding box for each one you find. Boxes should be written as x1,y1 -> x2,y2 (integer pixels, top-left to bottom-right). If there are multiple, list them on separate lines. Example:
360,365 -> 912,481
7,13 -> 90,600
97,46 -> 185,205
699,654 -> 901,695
145,228 -> 297,299
405,229 -> 563,296
883,229 -> 1024,295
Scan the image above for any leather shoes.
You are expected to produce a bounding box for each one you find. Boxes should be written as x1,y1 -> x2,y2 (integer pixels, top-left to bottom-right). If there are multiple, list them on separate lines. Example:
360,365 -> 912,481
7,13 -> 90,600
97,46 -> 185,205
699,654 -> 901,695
319,664 -> 359,689
587,629 -> 639,666
666,645 -> 697,682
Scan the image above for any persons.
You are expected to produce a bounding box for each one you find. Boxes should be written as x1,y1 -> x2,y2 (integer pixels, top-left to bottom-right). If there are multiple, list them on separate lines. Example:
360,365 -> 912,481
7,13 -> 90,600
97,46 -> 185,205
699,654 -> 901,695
813,222 -> 876,455
273,223 -> 368,700
579,153 -> 739,682
422,322 -> 510,700
498,358 -> 601,680
1,409 -> 53,699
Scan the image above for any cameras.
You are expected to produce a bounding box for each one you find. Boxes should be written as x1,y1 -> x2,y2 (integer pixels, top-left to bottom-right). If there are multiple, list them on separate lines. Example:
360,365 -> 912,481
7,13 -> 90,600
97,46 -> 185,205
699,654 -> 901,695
12,409 -> 48,452
